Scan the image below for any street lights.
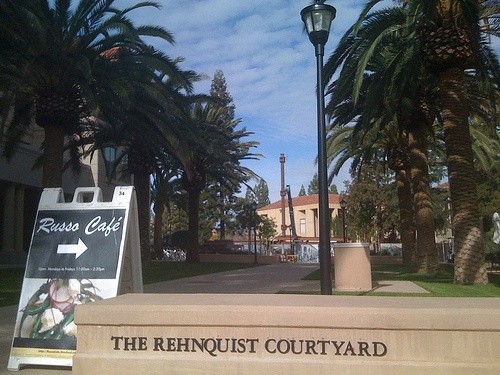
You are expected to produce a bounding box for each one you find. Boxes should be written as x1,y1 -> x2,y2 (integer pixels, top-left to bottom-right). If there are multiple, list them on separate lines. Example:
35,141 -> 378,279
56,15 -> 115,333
339,199 -> 349,242
299,0 -> 338,295
